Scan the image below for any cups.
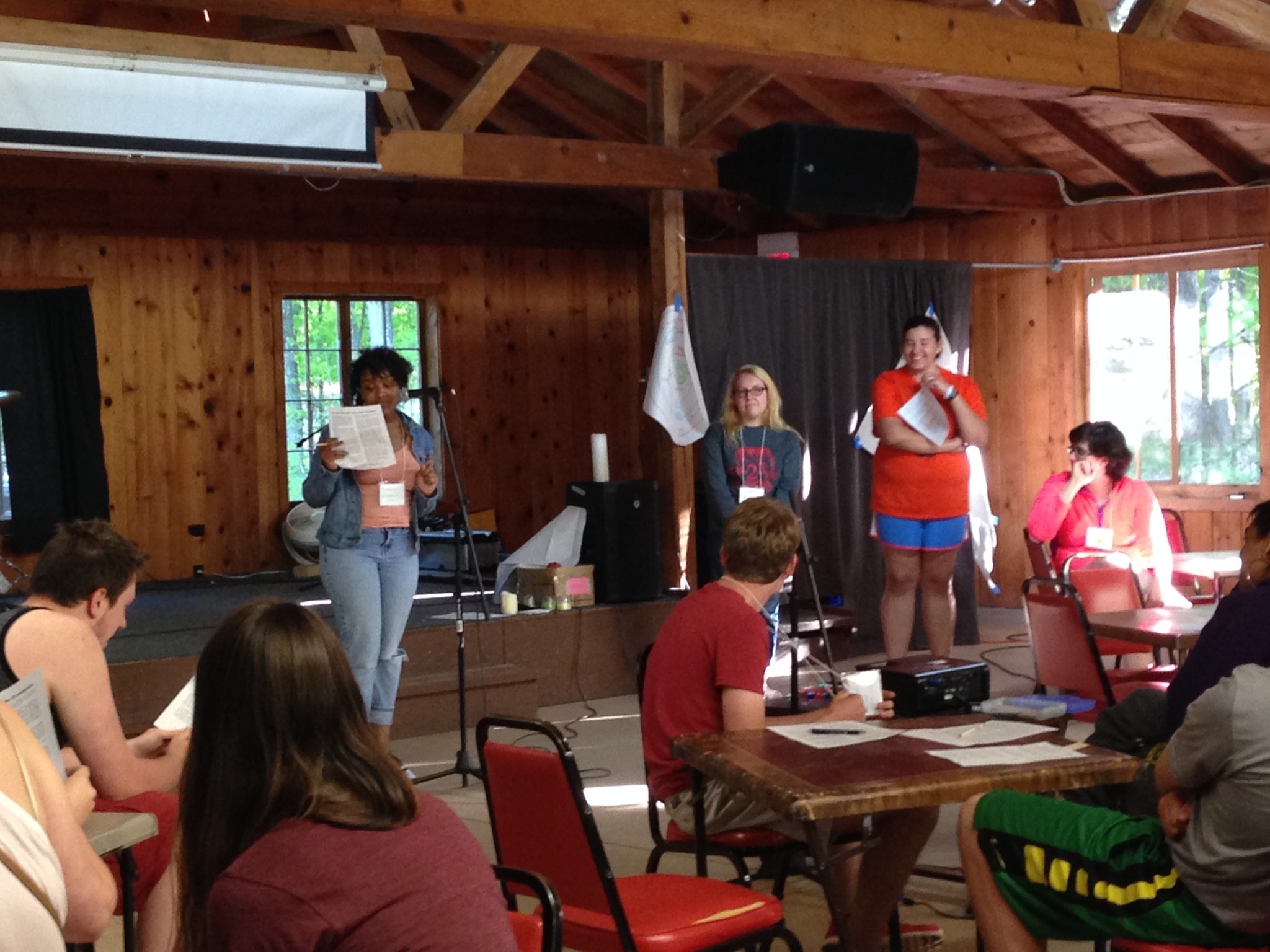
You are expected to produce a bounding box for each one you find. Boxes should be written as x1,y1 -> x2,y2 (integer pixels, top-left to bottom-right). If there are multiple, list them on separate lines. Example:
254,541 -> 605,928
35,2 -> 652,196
554,575 -> 572,611
500,576 -> 520,615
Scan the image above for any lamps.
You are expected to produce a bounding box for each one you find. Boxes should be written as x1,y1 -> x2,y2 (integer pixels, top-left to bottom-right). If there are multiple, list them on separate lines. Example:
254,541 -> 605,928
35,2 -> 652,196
280,501 -> 327,579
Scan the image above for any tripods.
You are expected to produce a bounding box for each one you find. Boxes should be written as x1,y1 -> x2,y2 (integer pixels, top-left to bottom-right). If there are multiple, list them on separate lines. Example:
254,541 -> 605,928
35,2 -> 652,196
411,400 -> 483,785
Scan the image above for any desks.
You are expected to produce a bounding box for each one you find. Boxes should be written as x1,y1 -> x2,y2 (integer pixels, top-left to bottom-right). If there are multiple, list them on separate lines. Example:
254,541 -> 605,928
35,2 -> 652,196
75,812 -> 159,952
671,715 -> 1144,952
1173,551 -> 1242,603
1088,604 -> 1218,665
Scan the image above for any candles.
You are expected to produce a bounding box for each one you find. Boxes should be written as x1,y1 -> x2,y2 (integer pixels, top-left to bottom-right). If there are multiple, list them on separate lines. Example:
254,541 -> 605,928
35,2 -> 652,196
591,433 -> 609,482
500,590 -> 518,615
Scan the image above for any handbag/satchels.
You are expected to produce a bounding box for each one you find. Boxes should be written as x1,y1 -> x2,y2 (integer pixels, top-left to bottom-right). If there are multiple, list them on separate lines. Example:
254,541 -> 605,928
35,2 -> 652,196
1094,688 -> 1173,755
566,479 -> 667,600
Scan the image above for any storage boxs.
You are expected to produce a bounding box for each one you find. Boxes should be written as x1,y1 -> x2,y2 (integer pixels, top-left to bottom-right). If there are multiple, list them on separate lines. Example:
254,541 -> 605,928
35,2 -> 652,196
420,530 -> 498,570
517,565 -> 595,607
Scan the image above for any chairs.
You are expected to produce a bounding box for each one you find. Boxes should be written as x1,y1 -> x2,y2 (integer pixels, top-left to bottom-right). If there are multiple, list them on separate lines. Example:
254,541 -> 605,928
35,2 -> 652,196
490,863 -> 562,952
1019,508 -> 1225,723
476,714 -> 784,952
639,644 -> 903,952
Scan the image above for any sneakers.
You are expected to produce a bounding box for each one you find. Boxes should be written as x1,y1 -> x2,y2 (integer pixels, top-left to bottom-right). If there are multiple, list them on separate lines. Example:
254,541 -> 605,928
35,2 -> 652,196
820,915 -> 943,952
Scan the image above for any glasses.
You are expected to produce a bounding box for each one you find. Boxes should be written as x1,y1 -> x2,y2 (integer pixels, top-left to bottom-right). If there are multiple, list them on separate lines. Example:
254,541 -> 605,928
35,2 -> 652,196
733,384 -> 768,397
1067,446 -> 1097,459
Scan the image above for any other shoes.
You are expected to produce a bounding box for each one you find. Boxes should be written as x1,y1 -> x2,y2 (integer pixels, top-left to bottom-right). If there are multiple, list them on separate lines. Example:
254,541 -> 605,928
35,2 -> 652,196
391,755 -> 416,780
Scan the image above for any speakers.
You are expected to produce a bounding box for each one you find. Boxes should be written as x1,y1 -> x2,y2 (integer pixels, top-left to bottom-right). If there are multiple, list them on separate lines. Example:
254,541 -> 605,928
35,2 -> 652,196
737,117 -> 921,217
568,479 -> 661,603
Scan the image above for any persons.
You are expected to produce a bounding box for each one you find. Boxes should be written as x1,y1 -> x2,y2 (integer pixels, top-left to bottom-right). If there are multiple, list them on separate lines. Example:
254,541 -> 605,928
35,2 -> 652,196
1027,420 -> 1195,671
641,497 -> 944,952
304,346 -> 443,760
696,364 -> 802,703
871,315 -> 988,662
960,499 -> 1270,952
0,521 -> 518,952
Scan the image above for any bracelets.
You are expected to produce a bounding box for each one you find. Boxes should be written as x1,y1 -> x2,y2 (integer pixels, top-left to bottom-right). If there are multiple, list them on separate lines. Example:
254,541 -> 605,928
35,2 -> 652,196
943,385 -> 959,400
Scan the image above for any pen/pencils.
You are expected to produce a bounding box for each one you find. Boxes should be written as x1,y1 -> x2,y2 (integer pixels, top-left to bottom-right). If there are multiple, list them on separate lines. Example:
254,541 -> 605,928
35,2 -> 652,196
316,440 -> 338,446
812,730 -> 865,735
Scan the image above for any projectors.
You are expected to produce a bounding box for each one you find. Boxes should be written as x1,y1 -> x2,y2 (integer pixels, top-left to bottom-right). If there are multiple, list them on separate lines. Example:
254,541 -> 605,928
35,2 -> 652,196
856,655 -> 990,714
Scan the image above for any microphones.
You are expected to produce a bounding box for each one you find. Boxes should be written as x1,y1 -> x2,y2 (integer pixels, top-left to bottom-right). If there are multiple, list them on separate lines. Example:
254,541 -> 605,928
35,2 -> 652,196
397,384 -> 451,401
